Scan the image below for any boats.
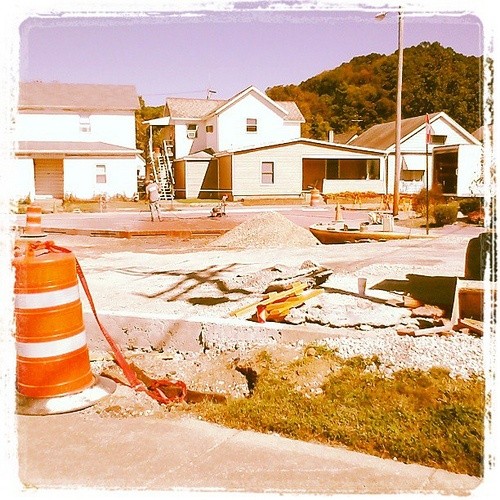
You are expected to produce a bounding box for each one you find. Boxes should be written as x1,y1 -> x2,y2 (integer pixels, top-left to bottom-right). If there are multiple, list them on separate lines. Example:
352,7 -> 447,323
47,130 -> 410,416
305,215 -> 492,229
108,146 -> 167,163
309,219 -> 442,244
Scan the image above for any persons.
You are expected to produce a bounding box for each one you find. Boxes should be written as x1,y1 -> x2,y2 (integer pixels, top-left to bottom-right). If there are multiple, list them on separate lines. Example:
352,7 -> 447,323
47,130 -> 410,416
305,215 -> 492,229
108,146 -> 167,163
143,180 -> 164,223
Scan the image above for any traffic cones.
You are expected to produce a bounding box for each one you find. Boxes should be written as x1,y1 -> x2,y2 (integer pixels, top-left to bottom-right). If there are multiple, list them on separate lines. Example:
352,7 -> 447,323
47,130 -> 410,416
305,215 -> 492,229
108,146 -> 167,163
332,201 -> 347,222
